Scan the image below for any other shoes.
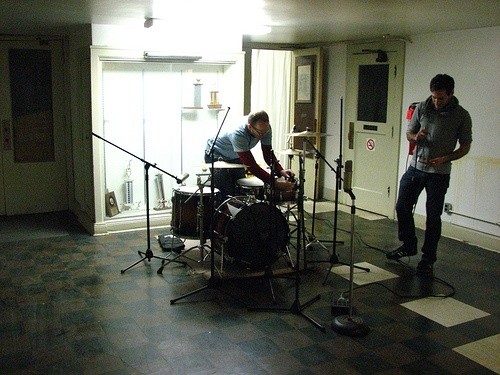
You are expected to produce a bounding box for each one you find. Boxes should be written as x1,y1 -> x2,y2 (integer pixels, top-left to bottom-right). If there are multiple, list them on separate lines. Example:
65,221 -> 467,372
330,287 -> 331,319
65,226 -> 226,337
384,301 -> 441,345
386,245 -> 417,259
418,261 -> 433,272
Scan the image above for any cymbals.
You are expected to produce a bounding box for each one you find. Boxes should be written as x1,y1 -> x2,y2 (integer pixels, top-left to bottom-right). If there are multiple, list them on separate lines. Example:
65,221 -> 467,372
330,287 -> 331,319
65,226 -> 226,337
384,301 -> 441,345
274,149 -> 310,155
283,131 -> 332,136
202,161 -> 250,168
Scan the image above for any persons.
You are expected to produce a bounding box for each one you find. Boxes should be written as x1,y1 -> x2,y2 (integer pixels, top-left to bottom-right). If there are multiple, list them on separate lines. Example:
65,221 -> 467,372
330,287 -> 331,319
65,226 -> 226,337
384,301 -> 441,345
203,111 -> 296,197
386,74 -> 472,274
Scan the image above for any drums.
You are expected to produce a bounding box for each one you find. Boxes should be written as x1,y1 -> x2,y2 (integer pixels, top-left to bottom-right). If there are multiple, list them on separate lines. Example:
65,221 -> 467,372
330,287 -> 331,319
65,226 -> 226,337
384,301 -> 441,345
234,177 -> 265,201
209,195 -> 290,269
169,185 -> 222,240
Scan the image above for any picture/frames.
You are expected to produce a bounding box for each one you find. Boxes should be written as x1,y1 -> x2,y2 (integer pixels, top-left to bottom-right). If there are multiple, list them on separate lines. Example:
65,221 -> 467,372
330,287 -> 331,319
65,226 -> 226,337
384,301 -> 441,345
296,64 -> 312,103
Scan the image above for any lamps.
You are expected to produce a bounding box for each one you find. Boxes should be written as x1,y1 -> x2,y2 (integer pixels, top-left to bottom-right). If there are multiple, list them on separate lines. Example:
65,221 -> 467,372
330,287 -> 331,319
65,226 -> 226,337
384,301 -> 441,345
144,51 -> 202,62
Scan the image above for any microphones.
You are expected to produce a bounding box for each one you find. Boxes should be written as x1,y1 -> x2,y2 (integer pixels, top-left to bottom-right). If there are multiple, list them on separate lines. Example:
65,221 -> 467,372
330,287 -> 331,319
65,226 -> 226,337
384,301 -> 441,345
287,125 -> 296,142
343,160 -> 353,191
177,173 -> 189,184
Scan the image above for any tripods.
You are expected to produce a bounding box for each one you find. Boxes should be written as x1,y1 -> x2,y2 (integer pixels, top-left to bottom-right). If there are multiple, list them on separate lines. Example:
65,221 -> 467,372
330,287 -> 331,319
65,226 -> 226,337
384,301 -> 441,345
91,96 -> 370,332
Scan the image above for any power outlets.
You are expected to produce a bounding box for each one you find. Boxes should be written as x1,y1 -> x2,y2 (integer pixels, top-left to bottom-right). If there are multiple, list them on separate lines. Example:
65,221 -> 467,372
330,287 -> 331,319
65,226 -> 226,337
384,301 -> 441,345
444,203 -> 452,214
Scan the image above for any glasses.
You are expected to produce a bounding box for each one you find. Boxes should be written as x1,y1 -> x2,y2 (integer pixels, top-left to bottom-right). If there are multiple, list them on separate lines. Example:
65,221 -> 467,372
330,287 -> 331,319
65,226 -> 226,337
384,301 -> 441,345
250,125 -> 271,135
